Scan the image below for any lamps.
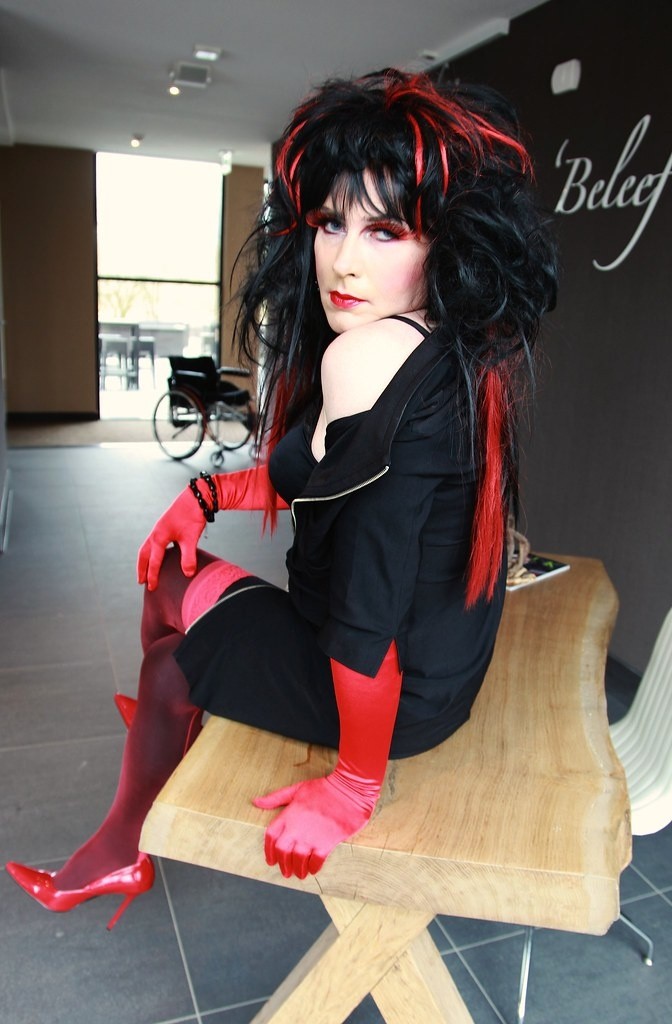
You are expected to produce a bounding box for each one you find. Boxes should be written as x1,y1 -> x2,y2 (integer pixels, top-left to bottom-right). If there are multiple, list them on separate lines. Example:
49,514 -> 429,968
192,43 -> 221,60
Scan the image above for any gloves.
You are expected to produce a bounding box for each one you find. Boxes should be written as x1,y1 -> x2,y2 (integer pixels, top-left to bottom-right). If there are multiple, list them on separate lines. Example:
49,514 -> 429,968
138,464 -> 290,591
252,639 -> 403,881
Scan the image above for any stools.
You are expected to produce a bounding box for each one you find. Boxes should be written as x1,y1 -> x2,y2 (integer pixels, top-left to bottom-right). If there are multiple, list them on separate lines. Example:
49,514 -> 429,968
100,334 -> 154,390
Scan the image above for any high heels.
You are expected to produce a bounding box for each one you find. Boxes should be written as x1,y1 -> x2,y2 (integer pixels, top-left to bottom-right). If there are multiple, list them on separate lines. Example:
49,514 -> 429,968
5,854 -> 155,931
114,695 -> 138,730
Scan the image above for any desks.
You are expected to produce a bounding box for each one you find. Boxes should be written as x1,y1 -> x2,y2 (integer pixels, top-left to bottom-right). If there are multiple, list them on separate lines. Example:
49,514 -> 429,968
136,552 -> 632,1024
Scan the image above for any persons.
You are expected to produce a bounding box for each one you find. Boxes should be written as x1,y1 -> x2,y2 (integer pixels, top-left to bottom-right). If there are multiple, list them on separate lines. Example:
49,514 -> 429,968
7,65 -> 558,929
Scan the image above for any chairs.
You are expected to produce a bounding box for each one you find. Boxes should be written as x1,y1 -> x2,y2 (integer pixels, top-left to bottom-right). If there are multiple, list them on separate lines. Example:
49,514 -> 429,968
98,323 -> 140,389
516,603 -> 672,1022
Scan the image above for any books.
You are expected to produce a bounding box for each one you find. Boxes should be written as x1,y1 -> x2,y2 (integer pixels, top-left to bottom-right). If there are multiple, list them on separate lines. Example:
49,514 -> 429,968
504,547 -> 570,591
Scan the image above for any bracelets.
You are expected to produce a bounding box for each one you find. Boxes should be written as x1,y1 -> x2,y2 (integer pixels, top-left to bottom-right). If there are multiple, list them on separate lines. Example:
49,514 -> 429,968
189,471 -> 220,523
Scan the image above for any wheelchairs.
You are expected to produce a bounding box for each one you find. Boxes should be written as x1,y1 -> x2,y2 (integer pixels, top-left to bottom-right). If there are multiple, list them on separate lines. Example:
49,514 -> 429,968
152,354 -> 266,469
